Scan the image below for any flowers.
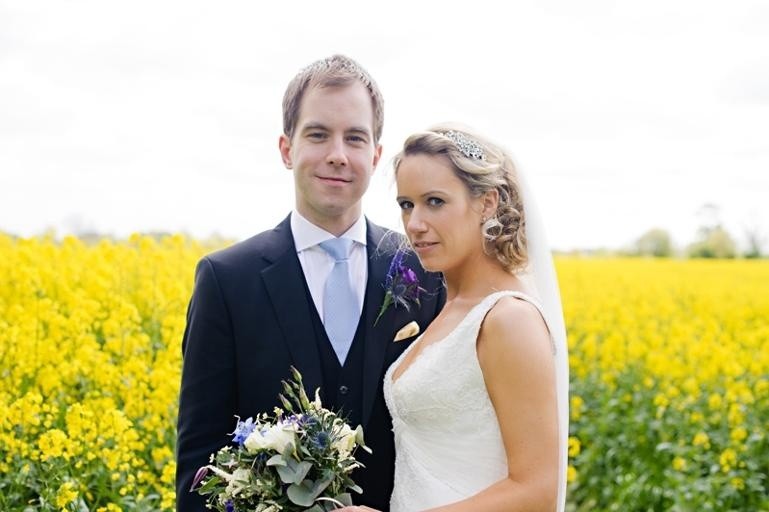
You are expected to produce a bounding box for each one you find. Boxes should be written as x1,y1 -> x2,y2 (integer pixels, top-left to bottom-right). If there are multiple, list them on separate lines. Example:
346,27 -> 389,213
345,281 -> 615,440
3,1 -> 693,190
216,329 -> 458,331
189,364 -> 372,511
374,267 -> 428,328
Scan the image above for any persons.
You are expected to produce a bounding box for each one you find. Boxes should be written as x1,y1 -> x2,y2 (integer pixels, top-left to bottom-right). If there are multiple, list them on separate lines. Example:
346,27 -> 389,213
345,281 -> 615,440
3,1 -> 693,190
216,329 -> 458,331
333,128 -> 571,511
175,54 -> 447,511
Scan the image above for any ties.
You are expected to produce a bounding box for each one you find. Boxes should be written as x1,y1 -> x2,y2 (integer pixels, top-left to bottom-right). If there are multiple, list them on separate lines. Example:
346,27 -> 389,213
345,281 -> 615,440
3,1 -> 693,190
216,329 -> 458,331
319,238 -> 360,367
